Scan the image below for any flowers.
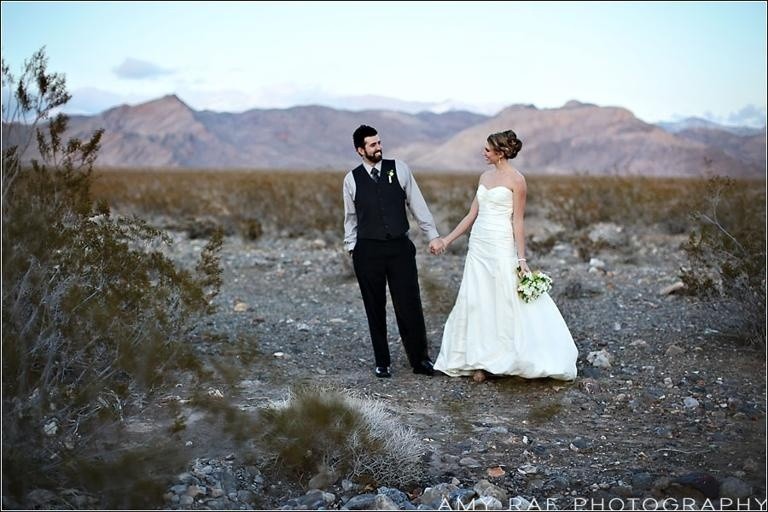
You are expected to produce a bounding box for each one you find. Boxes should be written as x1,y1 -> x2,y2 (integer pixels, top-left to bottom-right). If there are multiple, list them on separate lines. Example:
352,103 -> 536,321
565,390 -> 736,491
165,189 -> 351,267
515,267 -> 554,302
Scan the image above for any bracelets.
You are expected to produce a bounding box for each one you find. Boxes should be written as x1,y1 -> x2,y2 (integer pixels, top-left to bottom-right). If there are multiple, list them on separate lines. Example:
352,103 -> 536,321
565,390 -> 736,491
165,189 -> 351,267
517,258 -> 527,263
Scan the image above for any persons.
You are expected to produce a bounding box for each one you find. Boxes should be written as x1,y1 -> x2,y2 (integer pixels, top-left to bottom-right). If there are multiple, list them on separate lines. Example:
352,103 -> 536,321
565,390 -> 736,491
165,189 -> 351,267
342,124 -> 446,377
429,129 -> 579,384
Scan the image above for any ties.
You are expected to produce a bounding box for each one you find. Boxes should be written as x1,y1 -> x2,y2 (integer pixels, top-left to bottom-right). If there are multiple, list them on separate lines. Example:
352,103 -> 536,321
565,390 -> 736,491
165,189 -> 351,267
371,168 -> 380,183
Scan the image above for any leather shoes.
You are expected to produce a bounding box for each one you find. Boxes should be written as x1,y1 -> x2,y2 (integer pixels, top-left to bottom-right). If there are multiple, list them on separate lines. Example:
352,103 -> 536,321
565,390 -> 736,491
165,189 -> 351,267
473,370 -> 486,382
413,361 -> 442,376
376,365 -> 391,378
